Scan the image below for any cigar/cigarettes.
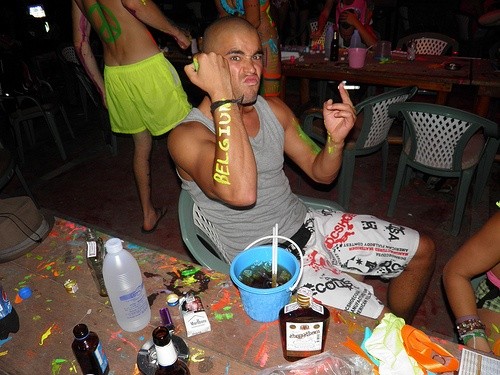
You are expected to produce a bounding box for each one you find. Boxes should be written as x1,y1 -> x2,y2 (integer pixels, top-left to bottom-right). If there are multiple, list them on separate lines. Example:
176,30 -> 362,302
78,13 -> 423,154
338,85 -> 359,89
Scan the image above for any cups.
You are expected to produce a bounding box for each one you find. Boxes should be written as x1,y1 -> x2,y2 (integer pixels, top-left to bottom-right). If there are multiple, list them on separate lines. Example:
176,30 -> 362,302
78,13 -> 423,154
376,41 -> 391,64
348,48 -> 366,68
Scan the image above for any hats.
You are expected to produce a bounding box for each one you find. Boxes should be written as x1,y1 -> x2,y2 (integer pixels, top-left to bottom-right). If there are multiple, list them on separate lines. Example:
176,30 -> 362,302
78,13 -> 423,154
0,196 -> 55,264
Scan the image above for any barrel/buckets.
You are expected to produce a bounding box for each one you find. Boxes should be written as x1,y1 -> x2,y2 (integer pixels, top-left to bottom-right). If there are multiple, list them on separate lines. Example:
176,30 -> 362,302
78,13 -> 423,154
230,236 -> 304,322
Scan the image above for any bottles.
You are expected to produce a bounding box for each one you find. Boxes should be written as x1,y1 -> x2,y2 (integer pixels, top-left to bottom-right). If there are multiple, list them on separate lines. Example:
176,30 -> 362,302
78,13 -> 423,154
0,283 -> 19,341
407,39 -> 416,63
71,324 -> 110,375
325,24 -> 333,58
85,227 -> 108,297
279,287 -> 329,362
330,32 -> 339,62
101,238 -> 151,332
152,326 -> 191,375
350,30 -> 361,48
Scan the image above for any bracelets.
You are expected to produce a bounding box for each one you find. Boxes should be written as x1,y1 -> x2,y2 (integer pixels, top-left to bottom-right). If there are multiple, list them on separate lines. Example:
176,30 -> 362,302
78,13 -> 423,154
210,99 -> 237,115
456,314 -> 489,344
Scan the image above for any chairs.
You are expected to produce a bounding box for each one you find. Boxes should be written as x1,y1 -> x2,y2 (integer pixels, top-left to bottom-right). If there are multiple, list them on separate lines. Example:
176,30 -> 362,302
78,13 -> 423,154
0,40 -> 119,203
179,17 -> 500,310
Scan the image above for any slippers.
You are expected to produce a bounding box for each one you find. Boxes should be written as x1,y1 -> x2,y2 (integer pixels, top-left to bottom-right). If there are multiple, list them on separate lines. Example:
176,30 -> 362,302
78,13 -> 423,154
141,207 -> 166,234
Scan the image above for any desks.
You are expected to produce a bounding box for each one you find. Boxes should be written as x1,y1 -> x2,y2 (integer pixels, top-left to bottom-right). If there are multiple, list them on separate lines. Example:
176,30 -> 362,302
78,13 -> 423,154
280,47 -> 470,115
470,59 -> 500,117
0,204 -> 499,375
90,44 -> 293,65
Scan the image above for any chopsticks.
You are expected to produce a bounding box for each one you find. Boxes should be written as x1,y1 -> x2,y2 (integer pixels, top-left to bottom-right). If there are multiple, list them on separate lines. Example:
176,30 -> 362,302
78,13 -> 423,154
272,222 -> 279,289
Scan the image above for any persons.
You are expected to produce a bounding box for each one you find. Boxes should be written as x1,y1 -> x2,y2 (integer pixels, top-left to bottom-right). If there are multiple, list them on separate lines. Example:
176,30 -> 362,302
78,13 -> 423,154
214,0 -> 283,96
71,1 -> 194,234
442,209 -> 500,358
0,25 -> 65,142
459,0 -> 500,118
270,0 -> 379,104
168,17 -> 436,322
